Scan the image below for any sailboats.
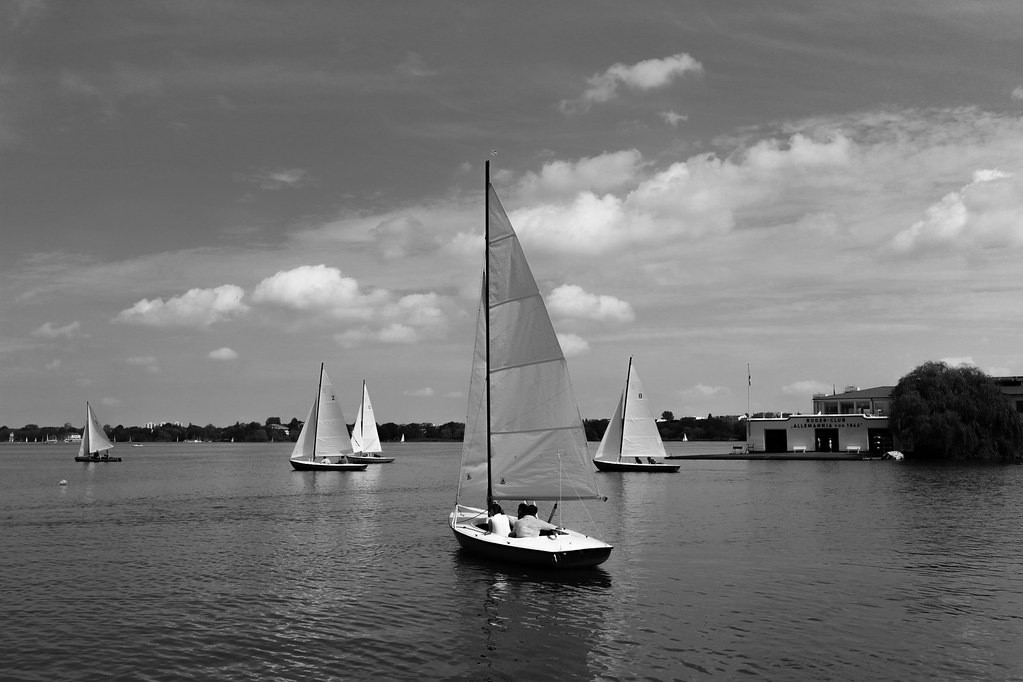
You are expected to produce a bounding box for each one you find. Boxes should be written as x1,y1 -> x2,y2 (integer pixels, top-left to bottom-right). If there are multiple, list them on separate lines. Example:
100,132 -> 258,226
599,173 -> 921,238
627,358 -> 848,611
346,379 -> 395,463
289,362 -> 368,470
592,354 -> 681,473
448,160 -> 613,568
683,433 -> 688,443
231,436 -> 234,443
271,438 -> 273,442
128,435 -> 132,444
113,435 -> 116,444
74,401 -> 122,462
400,433 -> 404,443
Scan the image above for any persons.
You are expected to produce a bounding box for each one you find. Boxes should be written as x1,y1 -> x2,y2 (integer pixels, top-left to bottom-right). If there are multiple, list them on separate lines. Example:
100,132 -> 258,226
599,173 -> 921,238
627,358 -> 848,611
509,503 -> 565,538
635,457 -> 642,464
366,453 -> 368,457
335,456 -> 346,464
488,504 -> 513,537
647,457 -> 656,464
104,449 -> 110,459
817,436 -> 821,451
321,456 -> 330,464
828,438 -> 832,453
93,450 -> 100,459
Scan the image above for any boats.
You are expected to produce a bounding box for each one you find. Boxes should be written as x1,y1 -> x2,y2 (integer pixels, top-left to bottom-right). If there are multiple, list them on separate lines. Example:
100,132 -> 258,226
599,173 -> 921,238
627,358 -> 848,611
183,437 -> 202,443
133,444 -> 144,446
